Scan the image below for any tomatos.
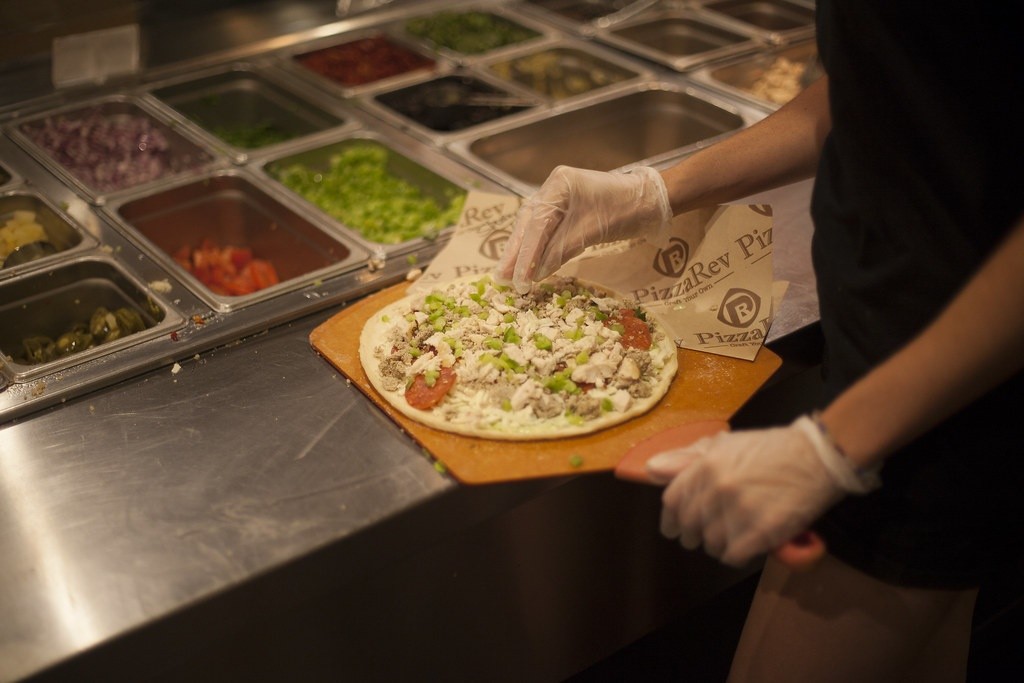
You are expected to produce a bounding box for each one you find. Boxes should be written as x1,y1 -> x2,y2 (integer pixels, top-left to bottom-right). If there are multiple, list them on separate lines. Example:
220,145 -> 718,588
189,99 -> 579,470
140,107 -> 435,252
172,240 -> 279,297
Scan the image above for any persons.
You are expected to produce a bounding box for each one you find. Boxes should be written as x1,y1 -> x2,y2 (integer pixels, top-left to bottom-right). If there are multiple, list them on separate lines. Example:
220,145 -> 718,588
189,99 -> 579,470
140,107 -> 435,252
502,1 -> 1024,683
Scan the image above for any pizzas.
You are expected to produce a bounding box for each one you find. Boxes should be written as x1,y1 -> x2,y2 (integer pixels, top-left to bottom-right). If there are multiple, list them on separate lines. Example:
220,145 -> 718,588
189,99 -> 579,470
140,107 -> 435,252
357,270 -> 679,440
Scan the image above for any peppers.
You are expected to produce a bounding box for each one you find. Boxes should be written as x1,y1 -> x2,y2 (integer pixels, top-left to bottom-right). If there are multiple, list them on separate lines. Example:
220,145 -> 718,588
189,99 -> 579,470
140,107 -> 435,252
12,306 -> 147,367
